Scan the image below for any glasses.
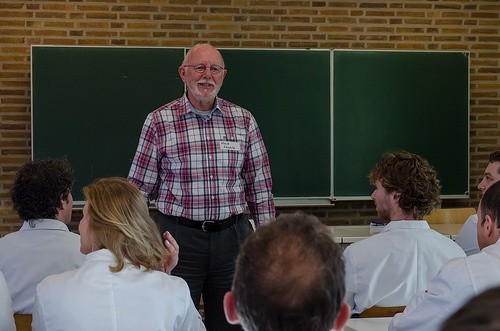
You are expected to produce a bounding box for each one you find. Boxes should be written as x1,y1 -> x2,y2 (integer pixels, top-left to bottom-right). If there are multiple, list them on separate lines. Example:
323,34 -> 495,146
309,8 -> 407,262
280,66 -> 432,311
184,63 -> 224,75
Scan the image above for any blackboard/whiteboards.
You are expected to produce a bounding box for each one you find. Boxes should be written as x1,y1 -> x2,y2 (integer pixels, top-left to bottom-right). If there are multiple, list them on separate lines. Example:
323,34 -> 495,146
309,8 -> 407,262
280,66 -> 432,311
29,44 -> 471,211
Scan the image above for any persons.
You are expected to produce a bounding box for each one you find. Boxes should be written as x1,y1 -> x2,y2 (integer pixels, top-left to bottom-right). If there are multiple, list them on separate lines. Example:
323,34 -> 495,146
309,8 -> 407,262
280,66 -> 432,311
128,43 -> 276,331
31,177 -> 207,331
0,270 -> 17,331
0,158 -> 86,315
342,150 -> 467,316
387,150 -> 500,331
223,210 -> 351,331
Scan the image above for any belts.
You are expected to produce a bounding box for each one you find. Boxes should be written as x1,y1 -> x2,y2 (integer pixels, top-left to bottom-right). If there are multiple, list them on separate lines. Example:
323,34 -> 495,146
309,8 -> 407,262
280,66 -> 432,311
158,211 -> 243,232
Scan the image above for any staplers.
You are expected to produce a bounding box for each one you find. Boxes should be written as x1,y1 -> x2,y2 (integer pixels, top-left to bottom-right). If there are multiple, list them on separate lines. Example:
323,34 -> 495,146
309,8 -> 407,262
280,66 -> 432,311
370,220 -> 384,226
